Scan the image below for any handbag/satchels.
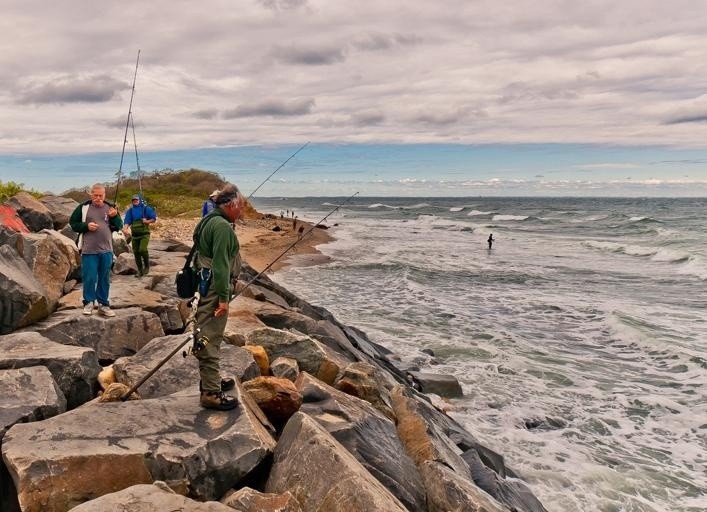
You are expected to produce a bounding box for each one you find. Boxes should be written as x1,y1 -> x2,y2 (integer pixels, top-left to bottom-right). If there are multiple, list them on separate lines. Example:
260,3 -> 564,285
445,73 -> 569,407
176,267 -> 195,298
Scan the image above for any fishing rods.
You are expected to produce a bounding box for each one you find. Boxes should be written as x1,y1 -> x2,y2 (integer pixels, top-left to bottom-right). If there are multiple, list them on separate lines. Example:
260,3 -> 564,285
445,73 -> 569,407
131,112 -> 144,231
113,48 -> 140,208
244,141 -> 310,203
121,190 -> 361,402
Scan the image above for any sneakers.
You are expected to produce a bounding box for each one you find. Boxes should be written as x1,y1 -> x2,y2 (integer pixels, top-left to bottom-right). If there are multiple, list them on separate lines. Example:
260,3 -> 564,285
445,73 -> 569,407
98,303 -> 115,317
200,378 -> 236,392
200,389 -> 238,410
83,302 -> 93,315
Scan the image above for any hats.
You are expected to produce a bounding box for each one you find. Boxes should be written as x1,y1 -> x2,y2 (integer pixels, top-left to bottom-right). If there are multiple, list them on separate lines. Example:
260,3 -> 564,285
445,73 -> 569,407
131,194 -> 140,200
209,189 -> 222,197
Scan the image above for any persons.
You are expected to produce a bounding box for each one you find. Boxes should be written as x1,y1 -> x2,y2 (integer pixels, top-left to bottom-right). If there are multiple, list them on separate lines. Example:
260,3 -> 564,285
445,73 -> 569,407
488,234 -> 493,250
194,184 -> 243,411
69,183 -> 123,317
280,209 -> 305,241
124,194 -> 155,278
